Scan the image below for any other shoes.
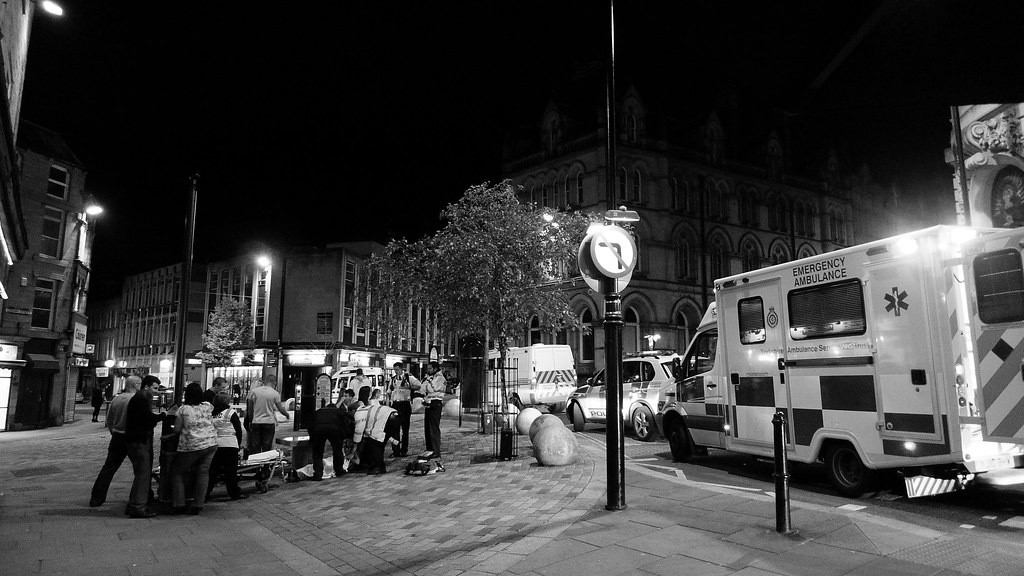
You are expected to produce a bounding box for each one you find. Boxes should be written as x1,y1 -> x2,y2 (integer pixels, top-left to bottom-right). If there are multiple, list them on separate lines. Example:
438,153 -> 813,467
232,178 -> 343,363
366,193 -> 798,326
92,419 -> 98,422
131,509 -> 156,518
311,476 -> 322,480
335,469 -> 346,475
89,500 -> 102,507
232,492 -> 250,499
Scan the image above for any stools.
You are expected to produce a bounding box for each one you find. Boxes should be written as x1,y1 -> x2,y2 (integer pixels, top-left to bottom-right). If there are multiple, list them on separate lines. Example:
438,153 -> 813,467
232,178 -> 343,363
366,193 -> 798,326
275,436 -> 310,469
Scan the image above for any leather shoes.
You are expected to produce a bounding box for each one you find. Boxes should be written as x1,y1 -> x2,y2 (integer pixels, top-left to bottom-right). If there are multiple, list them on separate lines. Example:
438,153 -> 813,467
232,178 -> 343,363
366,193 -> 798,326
428,451 -> 441,458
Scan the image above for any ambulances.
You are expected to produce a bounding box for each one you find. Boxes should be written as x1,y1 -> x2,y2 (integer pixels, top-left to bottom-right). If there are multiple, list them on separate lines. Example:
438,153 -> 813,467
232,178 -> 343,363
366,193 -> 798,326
660,225 -> 1024,499
485,343 -> 577,413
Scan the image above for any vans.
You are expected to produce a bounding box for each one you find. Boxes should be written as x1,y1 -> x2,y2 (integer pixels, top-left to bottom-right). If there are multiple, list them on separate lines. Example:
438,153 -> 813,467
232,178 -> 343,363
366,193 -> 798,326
330,366 -> 388,408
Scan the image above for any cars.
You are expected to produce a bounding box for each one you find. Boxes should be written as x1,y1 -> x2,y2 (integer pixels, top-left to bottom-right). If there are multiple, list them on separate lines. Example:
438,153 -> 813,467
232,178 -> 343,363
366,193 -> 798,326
565,351 -> 676,441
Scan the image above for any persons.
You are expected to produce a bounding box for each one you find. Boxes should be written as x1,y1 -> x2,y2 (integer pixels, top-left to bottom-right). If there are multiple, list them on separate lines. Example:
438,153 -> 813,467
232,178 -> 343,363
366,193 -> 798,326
244,374 -> 290,454
336,369 -> 382,413
212,391 -> 249,499
385,362 -> 421,458
288,445 -> 359,482
125,375 -> 168,517
308,403 -> 351,481
417,361 -> 447,460
349,403 -> 400,472
91,385 -> 103,422
167,383 -> 219,515
89,376 -> 160,508
203,377 -> 227,403
